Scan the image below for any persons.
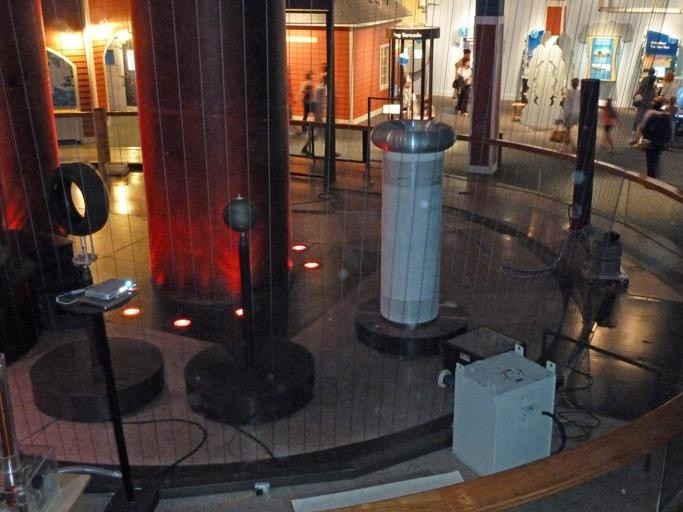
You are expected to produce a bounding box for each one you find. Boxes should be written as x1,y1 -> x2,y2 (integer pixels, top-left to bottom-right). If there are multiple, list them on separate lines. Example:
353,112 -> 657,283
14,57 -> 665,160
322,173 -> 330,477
301,61 -> 341,157
452,49 -> 473,116
402,76 -> 417,111
563,78 -> 581,131
628,68 -> 678,178
598,99 -> 623,153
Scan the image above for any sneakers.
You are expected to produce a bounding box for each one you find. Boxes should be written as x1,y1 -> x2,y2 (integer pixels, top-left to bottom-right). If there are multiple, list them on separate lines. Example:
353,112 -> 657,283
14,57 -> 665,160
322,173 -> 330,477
302,150 -> 315,155
336,152 -> 341,156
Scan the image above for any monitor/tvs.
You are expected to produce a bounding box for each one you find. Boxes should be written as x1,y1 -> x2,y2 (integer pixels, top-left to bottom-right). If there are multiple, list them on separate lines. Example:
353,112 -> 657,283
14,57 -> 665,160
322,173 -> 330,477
652,66 -> 666,83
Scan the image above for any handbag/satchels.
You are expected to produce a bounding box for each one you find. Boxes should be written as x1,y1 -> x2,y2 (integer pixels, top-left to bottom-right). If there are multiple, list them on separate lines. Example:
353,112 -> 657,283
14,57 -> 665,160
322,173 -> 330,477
633,137 -> 650,151
452,79 -> 466,89
632,94 -> 642,106
